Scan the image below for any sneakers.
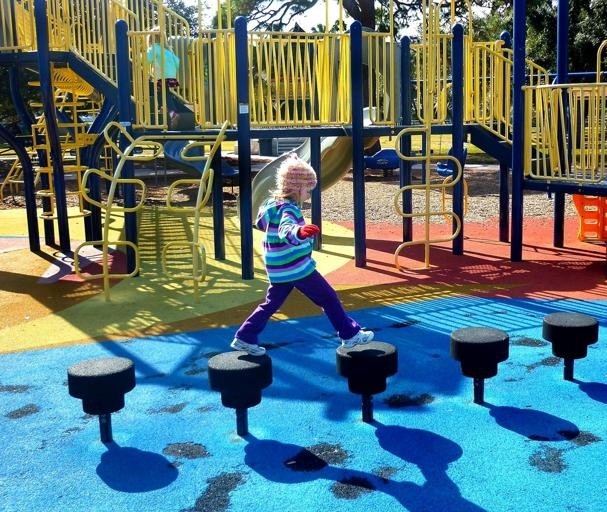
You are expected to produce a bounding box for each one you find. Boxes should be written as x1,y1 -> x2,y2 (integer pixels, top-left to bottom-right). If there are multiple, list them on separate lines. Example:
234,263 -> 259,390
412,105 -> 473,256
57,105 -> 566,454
231,337 -> 267,356
342,330 -> 375,349
170,112 -> 181,129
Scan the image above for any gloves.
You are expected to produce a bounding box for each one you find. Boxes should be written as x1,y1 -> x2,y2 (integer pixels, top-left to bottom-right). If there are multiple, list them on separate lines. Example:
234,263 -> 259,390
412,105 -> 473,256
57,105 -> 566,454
298,224 -> 321,238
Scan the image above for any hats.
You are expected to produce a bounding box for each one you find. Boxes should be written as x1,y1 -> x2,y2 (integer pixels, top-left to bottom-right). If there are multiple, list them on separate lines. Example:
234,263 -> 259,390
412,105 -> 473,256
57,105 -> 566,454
277,152 -> 318,201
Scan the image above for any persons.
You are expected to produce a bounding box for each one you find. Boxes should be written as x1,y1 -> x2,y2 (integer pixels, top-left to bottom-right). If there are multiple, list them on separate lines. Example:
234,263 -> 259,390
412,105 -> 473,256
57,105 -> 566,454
136,25 -> 179,127
228,152 -> 374,357
